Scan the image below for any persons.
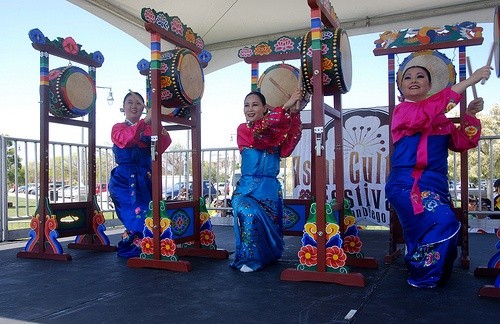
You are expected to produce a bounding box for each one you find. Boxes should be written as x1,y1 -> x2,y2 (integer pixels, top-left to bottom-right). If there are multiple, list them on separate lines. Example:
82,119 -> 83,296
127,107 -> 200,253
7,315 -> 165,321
232,89 -> 303,272
212,202 -> 234,218
385,65 -> 493,287
108,93 -> 172,257
468,179 -> 500,233
174,189 -> 191,200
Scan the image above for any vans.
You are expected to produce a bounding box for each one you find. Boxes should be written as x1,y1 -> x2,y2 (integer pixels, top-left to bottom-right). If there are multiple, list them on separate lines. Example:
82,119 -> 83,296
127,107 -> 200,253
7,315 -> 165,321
229,170 -> 241,201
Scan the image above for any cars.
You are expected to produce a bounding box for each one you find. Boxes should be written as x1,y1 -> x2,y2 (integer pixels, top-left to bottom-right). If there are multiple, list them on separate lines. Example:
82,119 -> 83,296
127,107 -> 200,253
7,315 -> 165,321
8,182 -> 115,211
446,179 -> 497,200
213,183 -> 228,196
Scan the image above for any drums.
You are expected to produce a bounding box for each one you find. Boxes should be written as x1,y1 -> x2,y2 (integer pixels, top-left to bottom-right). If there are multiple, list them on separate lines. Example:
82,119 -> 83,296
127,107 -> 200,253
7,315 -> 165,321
395,49 -> 457,101
493,5 -> 500,78
299,25 -> 353,94
146,47 -> 205,107
47,66 -> 96,119
252,64 -> 310,114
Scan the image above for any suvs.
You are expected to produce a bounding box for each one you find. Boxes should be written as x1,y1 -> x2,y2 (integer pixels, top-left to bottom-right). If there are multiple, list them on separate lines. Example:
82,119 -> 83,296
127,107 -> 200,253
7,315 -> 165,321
162,180 -> 217,205
